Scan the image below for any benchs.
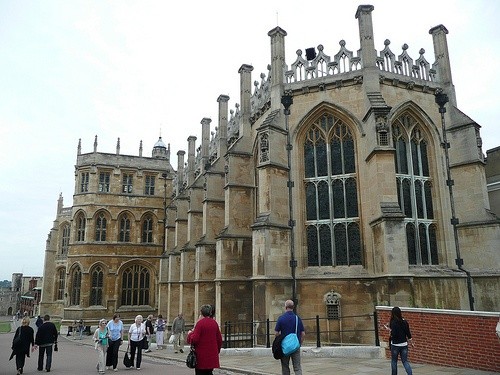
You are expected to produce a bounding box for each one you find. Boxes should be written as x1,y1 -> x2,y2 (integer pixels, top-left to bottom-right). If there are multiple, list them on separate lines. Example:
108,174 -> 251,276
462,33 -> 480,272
67,326 -> 91,336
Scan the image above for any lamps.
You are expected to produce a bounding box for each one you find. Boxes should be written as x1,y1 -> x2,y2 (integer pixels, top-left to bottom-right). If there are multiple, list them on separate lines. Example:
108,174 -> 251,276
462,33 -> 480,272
305,48 -> 316,61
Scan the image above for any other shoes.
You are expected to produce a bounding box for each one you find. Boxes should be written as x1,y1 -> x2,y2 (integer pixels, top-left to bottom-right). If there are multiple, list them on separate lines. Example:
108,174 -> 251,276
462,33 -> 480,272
112,367 -> 118,372
17,368 -> 23,375
180,349 -> 184,353
99,371 -> 105,374
131,366 -> 135,368
105,366 -> 110,370
174,352 -> 178,354
136,368 -> 140,370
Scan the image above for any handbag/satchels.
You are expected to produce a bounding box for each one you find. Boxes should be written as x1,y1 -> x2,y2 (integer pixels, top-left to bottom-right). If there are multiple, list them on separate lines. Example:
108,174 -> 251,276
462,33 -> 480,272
95,342 -> 98,350
13,335 -> 21,348
281,333 -> 301,356
142,336 -> 148,349
123,343 -> 132,368
186,342 -> 196,369
54,344 -> 58,351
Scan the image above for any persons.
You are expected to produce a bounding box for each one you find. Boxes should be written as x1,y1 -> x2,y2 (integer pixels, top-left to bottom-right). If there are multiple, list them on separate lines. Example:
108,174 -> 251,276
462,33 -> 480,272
274,299 -> 306,375
72,319 -> 84,339
11,316 -> 35,375
36,315 -> 43,327
187,304 -> 223,375
128,315 -> 146,370
92,319 -> 111,374
106,313 -> 124,372
144,315 -> 154,353
386,306 -> 417,375
171,313 -> 187,354
155,314 -> 166,350
34,315 -> 58,372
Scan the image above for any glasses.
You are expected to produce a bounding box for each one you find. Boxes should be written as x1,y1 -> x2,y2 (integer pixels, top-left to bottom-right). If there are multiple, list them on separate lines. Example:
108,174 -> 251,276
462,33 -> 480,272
102,323 -> 106,324
